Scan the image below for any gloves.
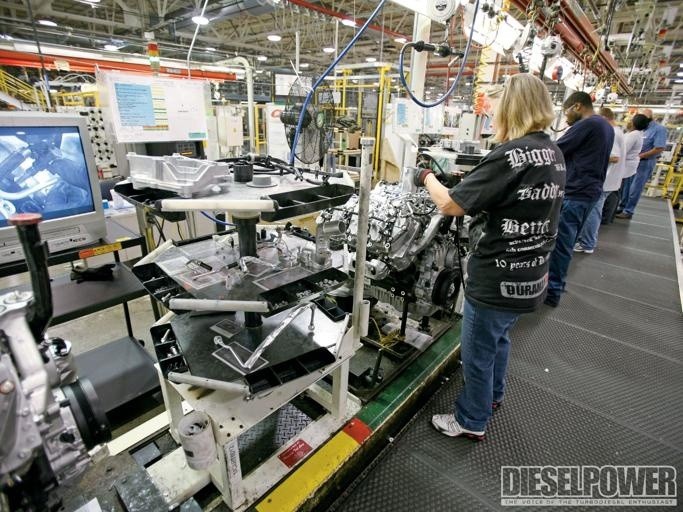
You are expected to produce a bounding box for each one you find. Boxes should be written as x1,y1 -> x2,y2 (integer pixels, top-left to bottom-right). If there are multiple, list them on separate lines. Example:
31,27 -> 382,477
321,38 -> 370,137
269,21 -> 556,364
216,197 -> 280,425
413,167 -> 432,187
435,174 -> 461,189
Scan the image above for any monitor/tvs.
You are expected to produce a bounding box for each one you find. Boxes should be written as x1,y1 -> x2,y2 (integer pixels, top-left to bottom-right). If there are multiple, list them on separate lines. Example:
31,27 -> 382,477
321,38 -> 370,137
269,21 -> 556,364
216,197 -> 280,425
0,111 -> 107,264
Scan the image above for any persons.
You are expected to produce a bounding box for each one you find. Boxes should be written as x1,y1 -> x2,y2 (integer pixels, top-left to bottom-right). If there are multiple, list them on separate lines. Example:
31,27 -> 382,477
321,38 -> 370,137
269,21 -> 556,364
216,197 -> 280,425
612,107 -> 668,220
571,107 -> 627,254
407,72 -> 567,443
542,90 -> 615,308
612,113 -> 649,214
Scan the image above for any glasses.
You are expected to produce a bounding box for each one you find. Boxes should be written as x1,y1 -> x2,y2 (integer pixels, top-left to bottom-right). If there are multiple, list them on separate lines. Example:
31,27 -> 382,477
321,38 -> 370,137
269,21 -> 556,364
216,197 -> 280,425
564,104 -> 575,115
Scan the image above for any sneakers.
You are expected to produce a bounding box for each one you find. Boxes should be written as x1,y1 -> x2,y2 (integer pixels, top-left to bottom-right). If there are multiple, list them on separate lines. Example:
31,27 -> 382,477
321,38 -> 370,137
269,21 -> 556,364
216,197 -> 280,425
615,212 -> 632,219
572,243 -> 593,253
432,414 -> 485,441
543,297 -> 558,308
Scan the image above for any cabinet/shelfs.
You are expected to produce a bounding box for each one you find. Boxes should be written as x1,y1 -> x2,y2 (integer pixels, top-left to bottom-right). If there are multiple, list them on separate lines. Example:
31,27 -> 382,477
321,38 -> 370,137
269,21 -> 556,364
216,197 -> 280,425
114,164 -> 365,511
0,217 -> 163,413
